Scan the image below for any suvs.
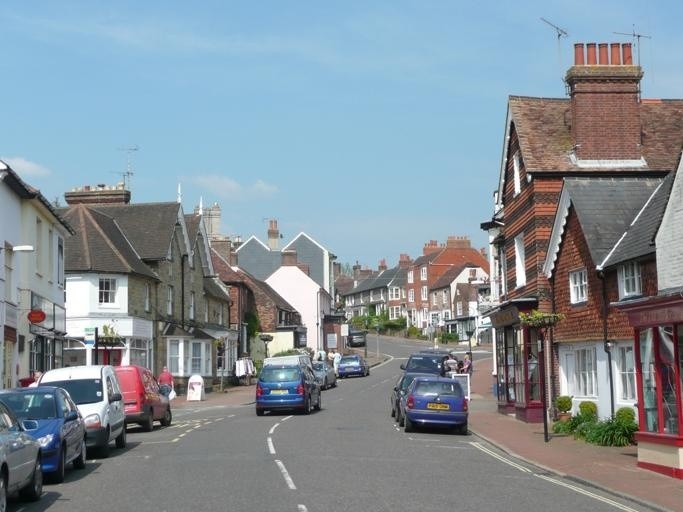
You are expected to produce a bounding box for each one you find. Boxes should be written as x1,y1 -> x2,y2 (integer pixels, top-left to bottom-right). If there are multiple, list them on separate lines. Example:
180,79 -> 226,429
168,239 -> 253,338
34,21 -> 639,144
348,329 -> 365,347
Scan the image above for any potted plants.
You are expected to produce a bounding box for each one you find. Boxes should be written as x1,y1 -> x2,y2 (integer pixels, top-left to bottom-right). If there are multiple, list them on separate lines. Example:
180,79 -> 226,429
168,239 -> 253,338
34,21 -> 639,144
553,395 -> 572,422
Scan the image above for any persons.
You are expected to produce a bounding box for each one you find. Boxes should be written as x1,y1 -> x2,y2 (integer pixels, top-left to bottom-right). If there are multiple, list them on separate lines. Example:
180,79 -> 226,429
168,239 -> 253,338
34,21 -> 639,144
447,355 -> 460,378
27,370 -> 42,388
460,354 -> 472,374
318,347 -> 342,378
157,365 -> 175,398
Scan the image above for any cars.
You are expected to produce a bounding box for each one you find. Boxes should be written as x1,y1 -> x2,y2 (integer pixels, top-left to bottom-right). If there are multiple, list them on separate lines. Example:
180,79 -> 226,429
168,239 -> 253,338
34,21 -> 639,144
0,387 -> 86,484
0,400 -> 44,512
658,389 -> 677,423
390,348 -> 471,436
254,355 -> 370,414
500,354 -> 512,376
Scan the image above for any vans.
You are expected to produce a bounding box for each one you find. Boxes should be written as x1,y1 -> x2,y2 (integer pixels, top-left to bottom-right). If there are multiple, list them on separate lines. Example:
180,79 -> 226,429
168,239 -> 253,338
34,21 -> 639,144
110,365 -> 173,433
26,365 -> 127,459
